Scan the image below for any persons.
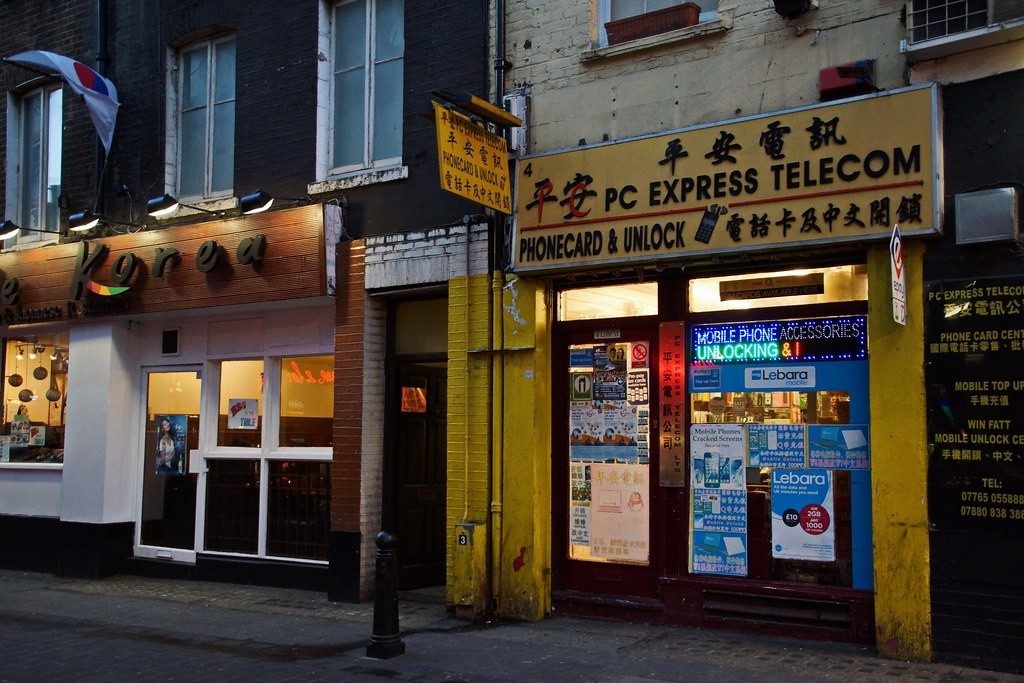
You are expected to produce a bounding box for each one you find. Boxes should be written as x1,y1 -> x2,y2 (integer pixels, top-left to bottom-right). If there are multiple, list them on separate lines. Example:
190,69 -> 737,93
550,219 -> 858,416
610,348 -> 623,360
159,419 -> 177,471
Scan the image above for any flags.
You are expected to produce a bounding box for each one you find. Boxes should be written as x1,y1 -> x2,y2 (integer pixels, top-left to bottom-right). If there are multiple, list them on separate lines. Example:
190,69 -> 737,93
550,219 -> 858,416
8,47 -> 119,158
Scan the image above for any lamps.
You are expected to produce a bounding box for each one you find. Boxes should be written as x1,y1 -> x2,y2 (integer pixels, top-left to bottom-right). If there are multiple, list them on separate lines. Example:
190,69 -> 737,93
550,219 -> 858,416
50,345 -> 58,360
29,344 -> 37,359
16,346 -> 25,360
